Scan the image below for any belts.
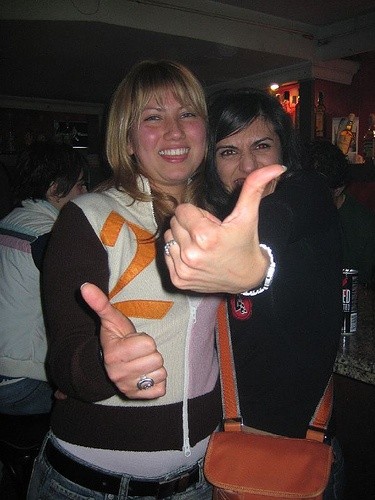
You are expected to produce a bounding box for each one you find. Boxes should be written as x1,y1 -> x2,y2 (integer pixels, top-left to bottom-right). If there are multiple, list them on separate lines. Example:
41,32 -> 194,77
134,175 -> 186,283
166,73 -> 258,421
41,433 -> 199,497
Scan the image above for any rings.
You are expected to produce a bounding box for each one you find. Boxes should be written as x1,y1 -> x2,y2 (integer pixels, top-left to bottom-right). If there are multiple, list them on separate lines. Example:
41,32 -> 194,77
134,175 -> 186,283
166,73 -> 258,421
137,374 -> 154,390
164,240 -> 176,255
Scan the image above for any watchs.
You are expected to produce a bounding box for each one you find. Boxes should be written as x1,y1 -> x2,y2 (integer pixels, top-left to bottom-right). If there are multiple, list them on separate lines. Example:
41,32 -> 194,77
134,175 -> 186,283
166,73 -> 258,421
240,244 -> 276,297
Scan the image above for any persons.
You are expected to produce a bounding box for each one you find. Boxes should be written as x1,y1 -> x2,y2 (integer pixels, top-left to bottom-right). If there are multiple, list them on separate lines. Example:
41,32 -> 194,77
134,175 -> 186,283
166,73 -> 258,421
1,59 -> 374,500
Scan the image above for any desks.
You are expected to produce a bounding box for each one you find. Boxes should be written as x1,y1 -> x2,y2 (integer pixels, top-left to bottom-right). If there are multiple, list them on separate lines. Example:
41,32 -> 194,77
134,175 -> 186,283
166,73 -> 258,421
333,283 -> 375,464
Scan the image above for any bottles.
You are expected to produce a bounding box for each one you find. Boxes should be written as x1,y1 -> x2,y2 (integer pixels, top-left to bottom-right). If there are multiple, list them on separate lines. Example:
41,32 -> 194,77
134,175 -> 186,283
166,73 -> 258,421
363,113 -> 375,164
276,91 -> 300,135
335,113 -> 355,157
315,92 -> 327,138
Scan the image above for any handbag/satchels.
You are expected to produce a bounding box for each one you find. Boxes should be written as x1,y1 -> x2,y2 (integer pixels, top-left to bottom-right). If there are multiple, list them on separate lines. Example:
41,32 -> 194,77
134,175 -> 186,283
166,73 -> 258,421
204,419 -> 335,500
